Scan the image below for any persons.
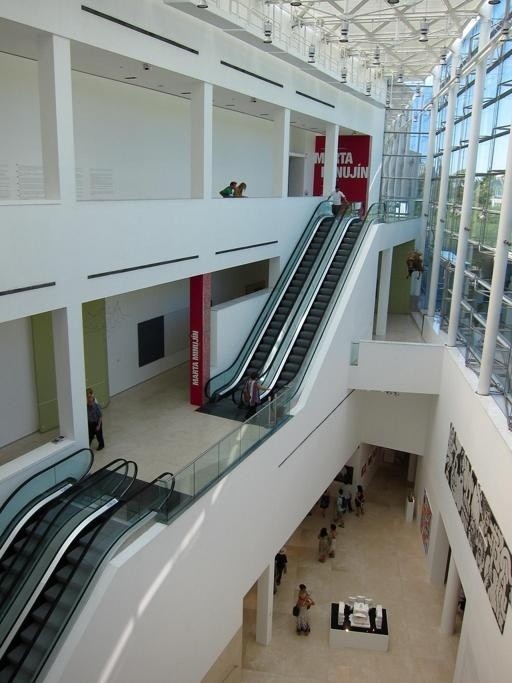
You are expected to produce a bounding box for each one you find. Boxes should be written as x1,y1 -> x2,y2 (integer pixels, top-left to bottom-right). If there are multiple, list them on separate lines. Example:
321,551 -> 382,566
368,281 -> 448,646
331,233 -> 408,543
275,547 -> 288,586
333,488 -> 347,528
457,592 -> 466,620
344,490 -> 353,513
329,523 -> 336,559
87,396 -> 104,451
327,185 -> 347,218
220,182 -> 237,197
319,490 -> 330,518
317,527 -> 330,563
233,183 -> 246,197
87,388 -> 97,404
241,371 -> 273,421
354,484 -> 368,517
296,584 -> 313,636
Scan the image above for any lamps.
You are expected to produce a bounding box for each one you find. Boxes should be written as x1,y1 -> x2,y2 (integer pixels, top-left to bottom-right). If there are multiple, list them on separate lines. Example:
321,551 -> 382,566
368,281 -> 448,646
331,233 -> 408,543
197,0 -> 209,8
262,1 -> 275,46
339,21 -> 348,44
498,20 -> 512,42
419,17 -> 428,42
307,44 -> 315,65
